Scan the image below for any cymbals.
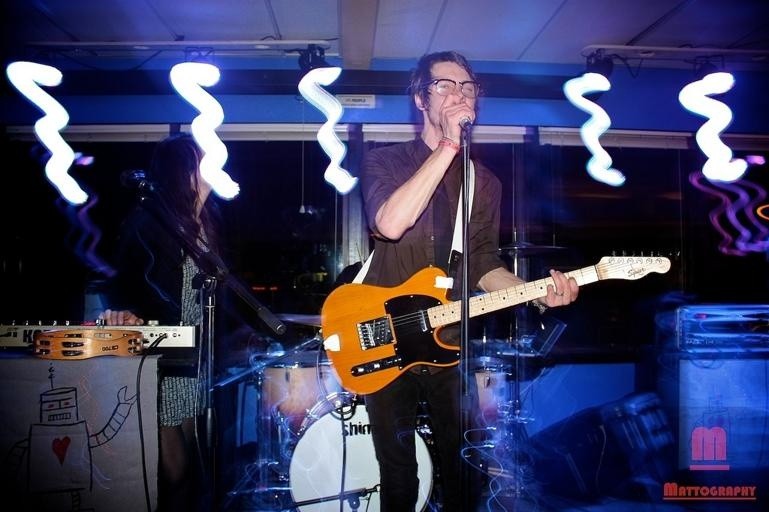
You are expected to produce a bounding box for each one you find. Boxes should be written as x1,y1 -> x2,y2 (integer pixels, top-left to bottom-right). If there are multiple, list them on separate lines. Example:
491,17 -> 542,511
279,311 -> 321,328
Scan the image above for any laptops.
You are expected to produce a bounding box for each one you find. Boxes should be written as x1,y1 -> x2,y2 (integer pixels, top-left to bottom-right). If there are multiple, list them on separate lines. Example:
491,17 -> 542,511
492,315 -> 568,357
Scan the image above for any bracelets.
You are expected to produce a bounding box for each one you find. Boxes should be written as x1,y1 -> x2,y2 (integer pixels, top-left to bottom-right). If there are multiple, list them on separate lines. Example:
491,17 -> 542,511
439,136 -> 460,152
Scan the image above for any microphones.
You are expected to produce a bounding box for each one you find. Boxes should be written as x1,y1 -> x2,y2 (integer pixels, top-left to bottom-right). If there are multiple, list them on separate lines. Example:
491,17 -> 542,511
120,168 -> 157,193
459,116 -> 472,131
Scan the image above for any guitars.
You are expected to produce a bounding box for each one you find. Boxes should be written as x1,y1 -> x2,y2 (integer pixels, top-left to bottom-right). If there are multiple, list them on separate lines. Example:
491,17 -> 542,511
321,252 -> 672,395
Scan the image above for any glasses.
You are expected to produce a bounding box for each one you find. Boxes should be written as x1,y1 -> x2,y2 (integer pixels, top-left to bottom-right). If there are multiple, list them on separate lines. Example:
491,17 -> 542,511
421,79 -> 481,97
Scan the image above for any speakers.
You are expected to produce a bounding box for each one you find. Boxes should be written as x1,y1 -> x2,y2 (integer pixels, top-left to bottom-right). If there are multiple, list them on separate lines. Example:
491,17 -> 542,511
518,390 -> 675,512
656,353 -> 769,506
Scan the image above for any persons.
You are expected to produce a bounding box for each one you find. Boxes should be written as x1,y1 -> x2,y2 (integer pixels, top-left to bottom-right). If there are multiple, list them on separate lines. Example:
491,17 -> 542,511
359,51 -> 578,512
98,132 -> 220,512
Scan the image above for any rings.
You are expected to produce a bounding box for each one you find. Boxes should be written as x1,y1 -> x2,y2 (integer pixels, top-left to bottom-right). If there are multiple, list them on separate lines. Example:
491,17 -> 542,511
556,293 -> 564,296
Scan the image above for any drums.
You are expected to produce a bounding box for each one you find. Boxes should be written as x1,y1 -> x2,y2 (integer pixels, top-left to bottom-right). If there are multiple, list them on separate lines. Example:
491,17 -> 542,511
249,351 -> 343,427
288,392 -> 436,511
467,363 -> 511,420
